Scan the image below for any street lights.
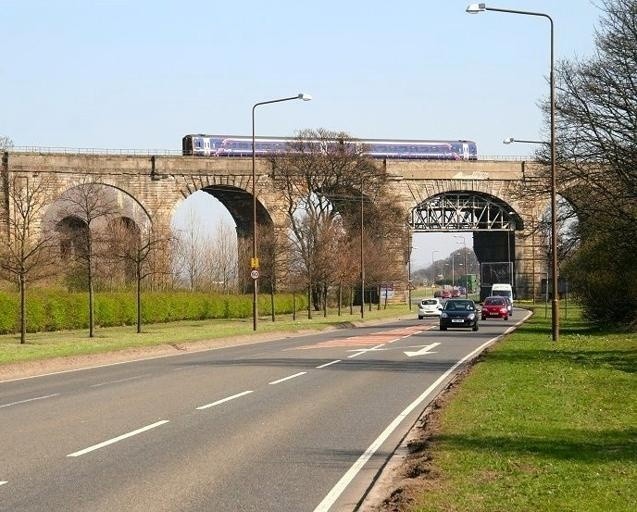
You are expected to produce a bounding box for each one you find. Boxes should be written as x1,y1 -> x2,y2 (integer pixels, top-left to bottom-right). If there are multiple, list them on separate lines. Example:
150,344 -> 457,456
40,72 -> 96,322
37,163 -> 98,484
509,209 -> 537,306
249,92 -> 313,333
463,0 -> 562,343
359,172 -> 407,320
503,134 -> 562,327
429,233 -> 480,289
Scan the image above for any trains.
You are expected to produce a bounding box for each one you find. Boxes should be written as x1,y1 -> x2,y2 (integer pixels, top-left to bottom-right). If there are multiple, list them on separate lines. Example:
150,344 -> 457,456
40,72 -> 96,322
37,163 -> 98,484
180,134 -> 478,166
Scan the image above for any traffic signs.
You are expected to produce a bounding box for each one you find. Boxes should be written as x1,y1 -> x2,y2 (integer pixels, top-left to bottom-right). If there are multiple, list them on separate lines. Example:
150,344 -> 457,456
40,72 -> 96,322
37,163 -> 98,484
250,269 -> 260,280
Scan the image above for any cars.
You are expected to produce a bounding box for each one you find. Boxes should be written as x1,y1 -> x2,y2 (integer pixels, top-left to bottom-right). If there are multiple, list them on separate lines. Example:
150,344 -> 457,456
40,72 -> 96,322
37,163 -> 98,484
418,273 -> 515,334
